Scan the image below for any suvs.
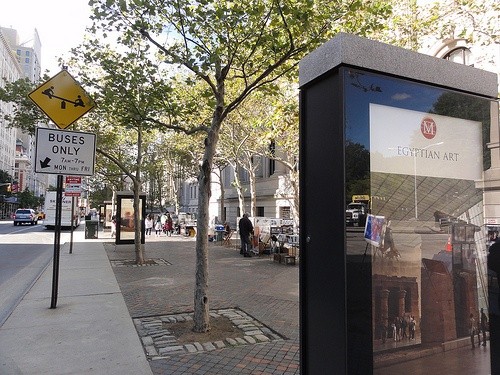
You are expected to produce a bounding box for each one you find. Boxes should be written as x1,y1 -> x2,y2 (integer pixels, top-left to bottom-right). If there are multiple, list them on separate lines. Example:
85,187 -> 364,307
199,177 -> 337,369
346,202 -> 369,226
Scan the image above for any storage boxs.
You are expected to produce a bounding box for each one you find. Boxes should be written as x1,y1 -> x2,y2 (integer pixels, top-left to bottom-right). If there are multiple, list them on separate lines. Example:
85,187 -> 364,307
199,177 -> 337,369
216,225 -> 224,230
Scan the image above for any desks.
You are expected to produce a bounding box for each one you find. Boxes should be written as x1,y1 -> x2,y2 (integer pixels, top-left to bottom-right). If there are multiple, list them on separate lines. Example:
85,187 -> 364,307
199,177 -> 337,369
214,230 -> 227,246
271,241 -> 289,264
289,243 -> 299,265
180,224 -> 197,237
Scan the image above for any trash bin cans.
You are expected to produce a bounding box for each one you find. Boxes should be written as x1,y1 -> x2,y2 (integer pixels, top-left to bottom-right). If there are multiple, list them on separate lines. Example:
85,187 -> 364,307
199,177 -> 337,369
85,220 -> 99,239
215,225 -> 224,241
85,215 -> 91,220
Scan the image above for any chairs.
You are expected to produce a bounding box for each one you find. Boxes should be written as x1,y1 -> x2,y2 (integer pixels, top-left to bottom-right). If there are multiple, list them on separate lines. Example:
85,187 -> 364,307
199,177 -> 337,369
221,230 -> 235,245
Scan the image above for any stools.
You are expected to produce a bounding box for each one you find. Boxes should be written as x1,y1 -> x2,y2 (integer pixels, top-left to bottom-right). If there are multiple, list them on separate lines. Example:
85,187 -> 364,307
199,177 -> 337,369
274,253 -> 295,266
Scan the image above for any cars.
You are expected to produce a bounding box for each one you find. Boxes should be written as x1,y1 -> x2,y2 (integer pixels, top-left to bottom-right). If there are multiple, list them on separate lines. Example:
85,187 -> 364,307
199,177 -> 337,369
35,211 -> 44,220
13,208 -> 39,225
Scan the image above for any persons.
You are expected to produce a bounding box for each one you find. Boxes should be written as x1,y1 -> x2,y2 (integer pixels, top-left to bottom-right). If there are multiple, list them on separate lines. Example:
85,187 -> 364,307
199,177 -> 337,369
161,211 -> 181,237
468,308 -> 488,349
489,230 -> 500,247
380,314 -> 416,340
223,223 -> 230,237
88,210 -> 100,227
239,212 -> 254,257
111,216 -> 117,239
145,214 -> 154,235
154,216 -> 162,235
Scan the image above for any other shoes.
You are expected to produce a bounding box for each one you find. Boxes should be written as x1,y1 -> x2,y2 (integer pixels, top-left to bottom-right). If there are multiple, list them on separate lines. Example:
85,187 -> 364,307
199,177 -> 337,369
244,253 -> 253,257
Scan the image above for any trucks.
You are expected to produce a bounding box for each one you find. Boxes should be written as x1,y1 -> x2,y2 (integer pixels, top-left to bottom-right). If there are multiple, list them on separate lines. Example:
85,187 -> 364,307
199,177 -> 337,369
44,188 -> 81,232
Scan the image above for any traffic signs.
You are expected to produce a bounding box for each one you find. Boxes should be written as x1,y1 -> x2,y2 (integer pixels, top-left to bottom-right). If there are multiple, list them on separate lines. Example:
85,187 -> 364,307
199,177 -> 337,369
34,126 -> 97,177
